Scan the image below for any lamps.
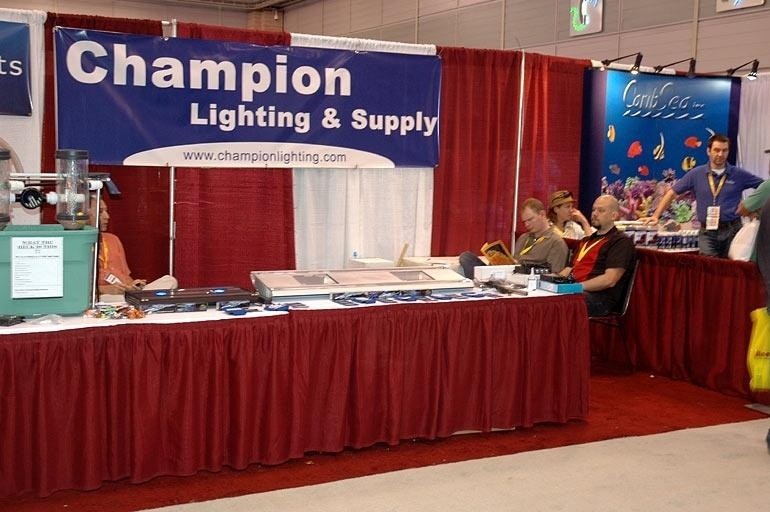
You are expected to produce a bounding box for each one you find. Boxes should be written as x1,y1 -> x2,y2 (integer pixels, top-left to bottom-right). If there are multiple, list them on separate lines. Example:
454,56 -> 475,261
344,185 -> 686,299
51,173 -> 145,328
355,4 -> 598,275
602,50 -> 644,77
654,58 -> 697,79
727,59 -> 760,81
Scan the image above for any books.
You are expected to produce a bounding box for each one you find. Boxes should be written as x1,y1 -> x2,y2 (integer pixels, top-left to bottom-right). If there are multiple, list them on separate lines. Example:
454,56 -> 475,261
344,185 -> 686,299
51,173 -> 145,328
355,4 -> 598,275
480,240 -> 518,264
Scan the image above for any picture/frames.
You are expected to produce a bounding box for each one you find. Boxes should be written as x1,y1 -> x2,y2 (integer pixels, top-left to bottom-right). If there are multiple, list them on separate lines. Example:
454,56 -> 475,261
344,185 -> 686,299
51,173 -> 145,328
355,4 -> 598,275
568,0 -> 604,38
715,0 -> 765,13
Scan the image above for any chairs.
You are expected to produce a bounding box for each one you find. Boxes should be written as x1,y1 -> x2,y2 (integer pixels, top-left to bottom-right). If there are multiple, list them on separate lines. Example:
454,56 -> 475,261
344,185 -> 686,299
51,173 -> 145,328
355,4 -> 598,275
583,250 -> 643,379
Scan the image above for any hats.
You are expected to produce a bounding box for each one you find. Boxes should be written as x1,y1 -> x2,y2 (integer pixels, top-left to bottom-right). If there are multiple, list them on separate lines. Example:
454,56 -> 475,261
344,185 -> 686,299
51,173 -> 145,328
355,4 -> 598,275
547,190 -> 578,210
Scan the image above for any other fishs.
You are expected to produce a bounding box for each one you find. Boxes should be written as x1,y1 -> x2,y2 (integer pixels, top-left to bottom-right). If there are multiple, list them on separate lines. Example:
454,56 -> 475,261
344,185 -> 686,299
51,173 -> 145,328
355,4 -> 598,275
651,131 -> 666,160
626,140 -> 643,159
682,136 -> 703,150
637,165 -> 650,177
609,163 -> 621,176
607,123 -> 616,143
680,155 -> 697,173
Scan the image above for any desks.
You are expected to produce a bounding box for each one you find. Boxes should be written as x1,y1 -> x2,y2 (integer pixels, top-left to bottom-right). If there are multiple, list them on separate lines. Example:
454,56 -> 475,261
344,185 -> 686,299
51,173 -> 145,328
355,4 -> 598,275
616,241 -> 770,404
0,268 -> 588,495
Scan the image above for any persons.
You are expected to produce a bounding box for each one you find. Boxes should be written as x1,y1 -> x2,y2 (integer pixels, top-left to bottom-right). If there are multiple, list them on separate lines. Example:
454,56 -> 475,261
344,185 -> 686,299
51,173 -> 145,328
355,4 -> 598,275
638,134 -> 765,260
87,195 -> 179,294
559,195 -> 637,316
548,189 -> 594,240
459,198 -> 569,281
736,148 -> 769,314
